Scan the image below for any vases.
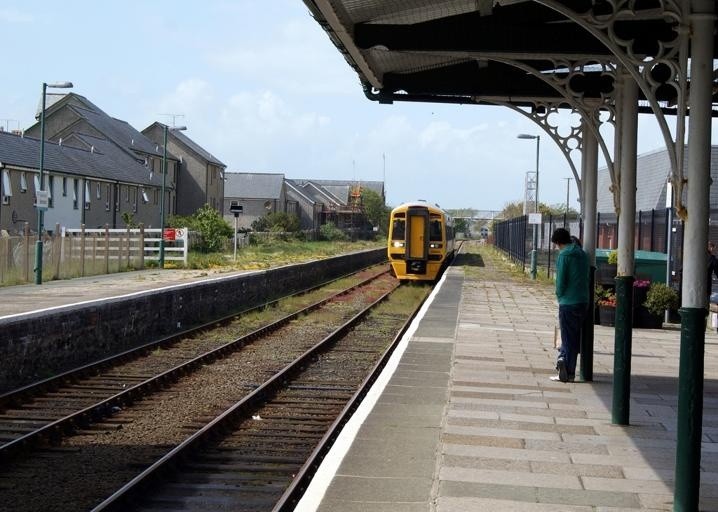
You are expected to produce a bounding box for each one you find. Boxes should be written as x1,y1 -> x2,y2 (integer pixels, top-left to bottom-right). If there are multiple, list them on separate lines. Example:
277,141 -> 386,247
599,306 -> 617,326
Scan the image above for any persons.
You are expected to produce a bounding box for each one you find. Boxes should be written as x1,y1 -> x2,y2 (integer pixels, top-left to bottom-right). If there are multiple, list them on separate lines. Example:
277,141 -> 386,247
549,236 -> 581,381
704,241 -> 717,327
549,228 -> 591,383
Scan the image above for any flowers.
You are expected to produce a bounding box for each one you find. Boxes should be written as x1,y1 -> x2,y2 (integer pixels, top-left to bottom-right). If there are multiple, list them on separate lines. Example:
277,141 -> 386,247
633,279 -> 650,288
598,288 -> 617,306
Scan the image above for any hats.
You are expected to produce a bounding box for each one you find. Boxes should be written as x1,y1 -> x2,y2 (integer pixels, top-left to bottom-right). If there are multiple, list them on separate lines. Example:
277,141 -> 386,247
552,228 -> 572,244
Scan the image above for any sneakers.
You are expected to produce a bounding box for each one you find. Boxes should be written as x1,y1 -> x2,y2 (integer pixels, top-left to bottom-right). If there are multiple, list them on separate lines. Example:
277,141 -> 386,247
550,375 -> 574,382
557,357 -> 568,382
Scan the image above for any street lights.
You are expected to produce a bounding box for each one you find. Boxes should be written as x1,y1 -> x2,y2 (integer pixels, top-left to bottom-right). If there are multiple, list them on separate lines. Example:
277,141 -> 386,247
33,81 -> 74,284
517,134 -> 540,280
158,124 -> 187,267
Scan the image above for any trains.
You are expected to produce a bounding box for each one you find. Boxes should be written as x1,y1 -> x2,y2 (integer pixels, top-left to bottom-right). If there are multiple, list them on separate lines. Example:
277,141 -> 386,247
387,200 -> 456,284
